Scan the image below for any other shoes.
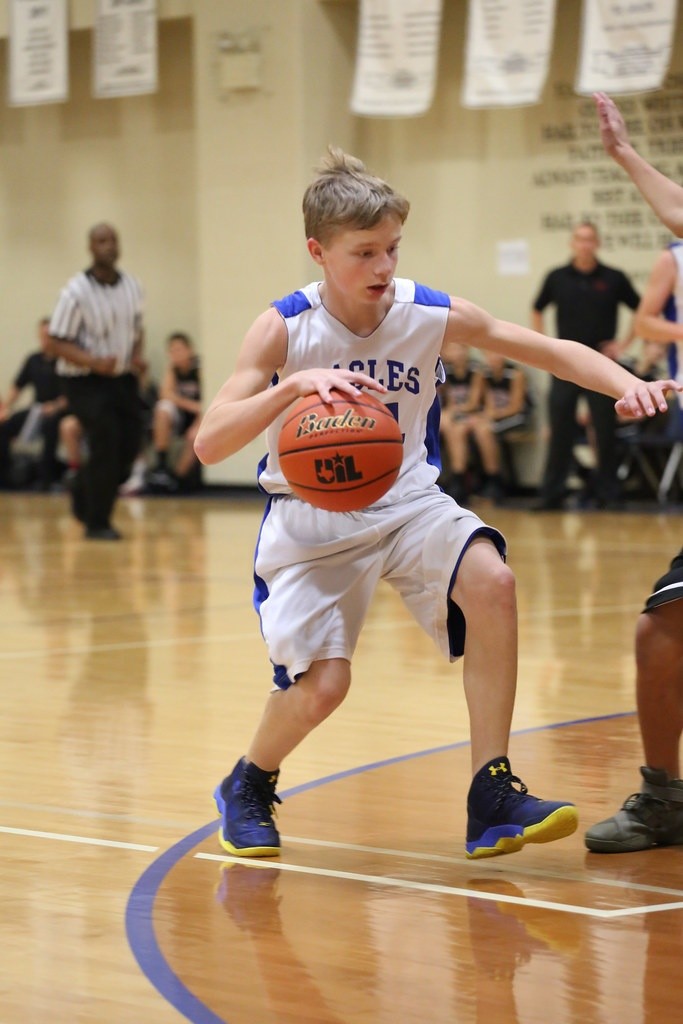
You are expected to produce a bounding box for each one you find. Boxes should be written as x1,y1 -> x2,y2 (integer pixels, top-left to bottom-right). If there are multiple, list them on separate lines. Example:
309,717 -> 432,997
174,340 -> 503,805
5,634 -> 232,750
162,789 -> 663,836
532,498 -> 564,510
70,481 -> 84,521
448,473 -> 468,504
485,473 -> 506,502
597,500 -> 626,510
87,528 -> 118,539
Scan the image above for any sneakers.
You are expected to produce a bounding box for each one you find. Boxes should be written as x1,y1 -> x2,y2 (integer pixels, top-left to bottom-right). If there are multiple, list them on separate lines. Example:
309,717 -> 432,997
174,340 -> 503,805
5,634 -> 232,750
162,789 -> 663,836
585,766 -> 683,850
214,755 -> 282,856
465,756 -> 579,858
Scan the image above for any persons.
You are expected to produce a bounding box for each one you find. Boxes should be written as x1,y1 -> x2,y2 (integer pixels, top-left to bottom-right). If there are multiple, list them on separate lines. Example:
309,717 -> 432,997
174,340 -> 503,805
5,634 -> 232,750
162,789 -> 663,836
583,87 -> 683,851
0,221 -> 203,542
192,151 -> 683,858
437,224 -> 683,510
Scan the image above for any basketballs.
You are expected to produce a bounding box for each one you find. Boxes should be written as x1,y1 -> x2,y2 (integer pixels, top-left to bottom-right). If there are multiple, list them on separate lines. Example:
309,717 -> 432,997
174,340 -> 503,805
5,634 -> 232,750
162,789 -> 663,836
279,388 -> 403,510
